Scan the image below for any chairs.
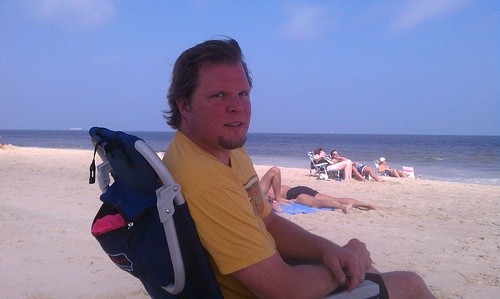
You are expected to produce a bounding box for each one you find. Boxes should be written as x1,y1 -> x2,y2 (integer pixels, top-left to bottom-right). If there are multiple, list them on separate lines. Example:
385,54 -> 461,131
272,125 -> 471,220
88,127 -> 380,299
374,160 -> 389,177
307,152 -> 339,180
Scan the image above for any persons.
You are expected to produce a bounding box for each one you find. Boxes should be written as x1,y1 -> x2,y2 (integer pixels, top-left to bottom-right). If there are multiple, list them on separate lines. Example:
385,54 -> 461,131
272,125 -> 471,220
259,166 -> 282,212
307,146 -> 413,184
280,185 -> 378,214
160,37 -> 437,299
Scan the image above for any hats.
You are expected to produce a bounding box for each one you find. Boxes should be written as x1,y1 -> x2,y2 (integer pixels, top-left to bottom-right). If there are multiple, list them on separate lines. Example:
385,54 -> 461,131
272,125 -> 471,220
380,157 -> 385,163
